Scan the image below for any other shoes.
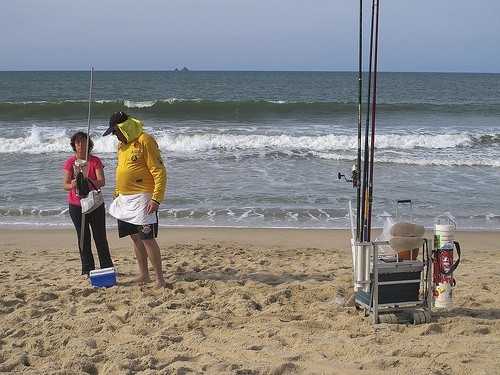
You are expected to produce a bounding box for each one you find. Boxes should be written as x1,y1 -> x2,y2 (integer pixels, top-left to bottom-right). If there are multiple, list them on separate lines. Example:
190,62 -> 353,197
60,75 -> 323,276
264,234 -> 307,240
390,221 -> 425,237
389,237 -> 423,252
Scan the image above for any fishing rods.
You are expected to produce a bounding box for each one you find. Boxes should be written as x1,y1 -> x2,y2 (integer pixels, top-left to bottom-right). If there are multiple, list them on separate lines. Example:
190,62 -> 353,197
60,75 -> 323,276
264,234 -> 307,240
362,1 -> 376,241
368,0 -> 380,242
356,1 -> 363,240
79,66 -> 94,253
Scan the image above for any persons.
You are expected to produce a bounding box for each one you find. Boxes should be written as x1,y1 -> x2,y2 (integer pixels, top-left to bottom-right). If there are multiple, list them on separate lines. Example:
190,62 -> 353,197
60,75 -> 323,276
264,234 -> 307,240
62,131 -> 114,281
102,112 -> 167,293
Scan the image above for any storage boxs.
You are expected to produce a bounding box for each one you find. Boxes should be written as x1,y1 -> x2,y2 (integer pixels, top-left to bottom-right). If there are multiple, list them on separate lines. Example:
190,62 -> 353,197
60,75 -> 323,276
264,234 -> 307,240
90,267 -> 116,288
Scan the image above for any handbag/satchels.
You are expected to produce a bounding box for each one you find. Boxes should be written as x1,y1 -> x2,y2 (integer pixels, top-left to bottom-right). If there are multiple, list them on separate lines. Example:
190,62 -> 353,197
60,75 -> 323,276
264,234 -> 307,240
78,177 -> 103,214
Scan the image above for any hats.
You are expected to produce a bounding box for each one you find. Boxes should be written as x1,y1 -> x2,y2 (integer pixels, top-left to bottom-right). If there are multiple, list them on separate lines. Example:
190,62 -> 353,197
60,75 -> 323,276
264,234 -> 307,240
101,112 -> 128,136
